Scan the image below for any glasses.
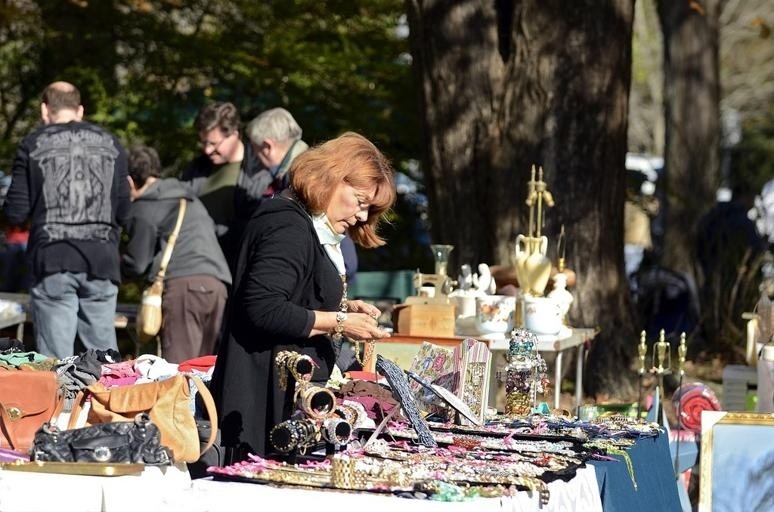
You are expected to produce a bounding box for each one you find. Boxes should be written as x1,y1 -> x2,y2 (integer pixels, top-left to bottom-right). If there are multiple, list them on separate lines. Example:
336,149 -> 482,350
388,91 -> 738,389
196,136 -> 227,149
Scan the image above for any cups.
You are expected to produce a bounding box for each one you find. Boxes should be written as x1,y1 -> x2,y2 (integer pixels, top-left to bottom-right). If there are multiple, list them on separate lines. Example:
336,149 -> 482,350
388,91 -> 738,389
523,297 -> 561,335
474,295 -> 517,338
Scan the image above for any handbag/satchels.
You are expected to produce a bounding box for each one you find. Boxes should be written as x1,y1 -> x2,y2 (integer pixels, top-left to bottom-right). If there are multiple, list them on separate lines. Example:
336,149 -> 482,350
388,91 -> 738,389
134,279 -> 164,344
0,370 -> 64,452
66,372 -> 218,464
29,412 -> 174,466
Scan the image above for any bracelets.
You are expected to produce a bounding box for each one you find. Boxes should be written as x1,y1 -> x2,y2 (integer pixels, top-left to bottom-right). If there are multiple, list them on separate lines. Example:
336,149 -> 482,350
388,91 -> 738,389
267,348 -> 358,452
327,310 -> 347,343
329,456 -> 358,491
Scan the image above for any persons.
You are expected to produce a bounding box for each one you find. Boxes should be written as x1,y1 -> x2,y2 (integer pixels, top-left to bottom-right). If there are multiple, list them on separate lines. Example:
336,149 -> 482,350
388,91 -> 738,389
245,104 -> 359,285
0,173 -> 32,289
121,145 -> 232,364
169,101 -> 270,290
212,133 -> 396,463
1,80 -> 131,358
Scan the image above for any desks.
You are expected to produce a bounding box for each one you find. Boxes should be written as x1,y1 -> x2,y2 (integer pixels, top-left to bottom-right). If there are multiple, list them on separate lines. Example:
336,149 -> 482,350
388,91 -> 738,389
0,291 -> 143,362
383,325 -> 600,409
193,412 -> 683,512
0,461 -> 193,512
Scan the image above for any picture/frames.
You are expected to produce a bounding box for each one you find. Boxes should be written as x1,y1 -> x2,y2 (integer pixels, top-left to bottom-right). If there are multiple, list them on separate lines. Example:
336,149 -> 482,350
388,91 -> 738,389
362,337 -> 490,376
698,410 -> 774,512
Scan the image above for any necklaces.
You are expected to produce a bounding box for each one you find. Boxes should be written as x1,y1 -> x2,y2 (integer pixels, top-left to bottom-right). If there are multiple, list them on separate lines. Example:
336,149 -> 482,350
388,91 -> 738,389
339,273 -> 350,313
238,458 -> 330,488
361,415 -> 666,505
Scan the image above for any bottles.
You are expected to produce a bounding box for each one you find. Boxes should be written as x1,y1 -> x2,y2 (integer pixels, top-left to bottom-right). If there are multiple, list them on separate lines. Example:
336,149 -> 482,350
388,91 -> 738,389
757,346 -> 774,411
744,379 -> 758,410
